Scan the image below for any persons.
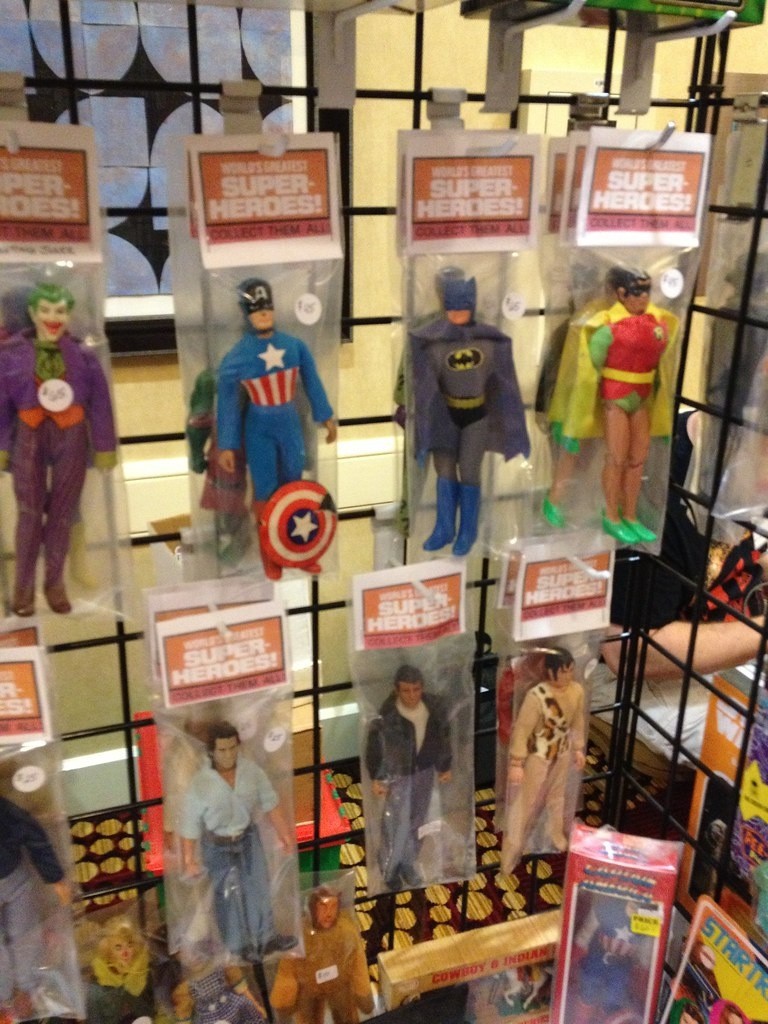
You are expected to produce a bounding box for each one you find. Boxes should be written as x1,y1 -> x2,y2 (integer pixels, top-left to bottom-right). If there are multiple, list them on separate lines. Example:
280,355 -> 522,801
152,921 -> 265,1024
365,665 -> 453,893
218,277 -> 338,581
269,887 -> 375,1023
0,283 -> 116,616
571,896 -> 650,1024
587,346 -> 768,852
0,795 -> 73,1024
82,924 -> 159,1024
536,266 -> 681,547
497,638 -> 588,873
392,267 -> 532,555
163,708 -> 298,964
186,367 -> 256,568
664,995 -> 750,1024
